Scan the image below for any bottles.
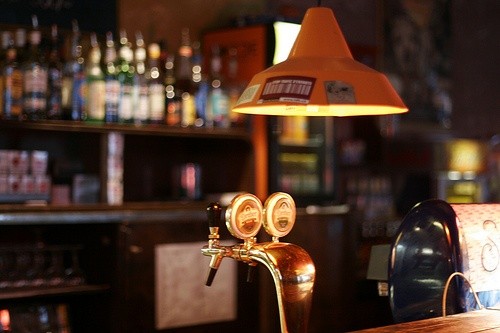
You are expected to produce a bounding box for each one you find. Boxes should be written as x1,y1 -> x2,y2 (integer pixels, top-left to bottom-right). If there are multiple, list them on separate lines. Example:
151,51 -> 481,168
1,10 -> 247,131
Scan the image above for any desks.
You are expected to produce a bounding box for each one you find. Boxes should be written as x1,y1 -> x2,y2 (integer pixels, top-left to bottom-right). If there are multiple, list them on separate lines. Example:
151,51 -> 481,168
344,308 -> 500,333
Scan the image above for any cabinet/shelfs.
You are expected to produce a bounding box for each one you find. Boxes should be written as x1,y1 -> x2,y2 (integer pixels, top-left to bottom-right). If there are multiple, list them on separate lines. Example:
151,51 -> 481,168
0,115 -> 259,332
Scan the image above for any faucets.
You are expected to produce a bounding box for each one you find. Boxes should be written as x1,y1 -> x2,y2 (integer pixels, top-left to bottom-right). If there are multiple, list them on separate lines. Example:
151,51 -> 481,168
201,245 -> 228,286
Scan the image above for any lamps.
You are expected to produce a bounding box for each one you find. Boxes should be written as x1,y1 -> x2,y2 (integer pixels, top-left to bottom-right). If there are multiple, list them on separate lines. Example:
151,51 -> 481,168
231,0 -> 408,119
